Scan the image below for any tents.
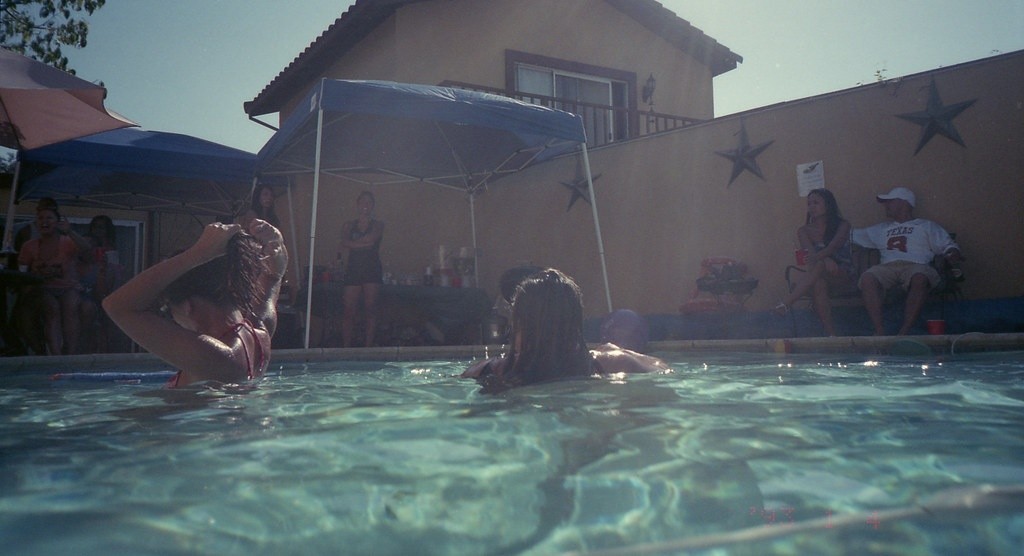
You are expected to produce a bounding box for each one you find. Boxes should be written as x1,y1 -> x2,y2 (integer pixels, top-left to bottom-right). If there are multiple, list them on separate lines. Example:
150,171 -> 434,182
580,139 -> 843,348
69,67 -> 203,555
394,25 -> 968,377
254,76 -> 617,349
0,127 -> 307,340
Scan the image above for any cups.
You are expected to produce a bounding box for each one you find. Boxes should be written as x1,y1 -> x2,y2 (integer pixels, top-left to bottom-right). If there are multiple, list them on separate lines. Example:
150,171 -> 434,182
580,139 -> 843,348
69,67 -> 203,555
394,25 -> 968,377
926,319 -> 946,335
794,249 -> 809,265
320,272 -> 330,280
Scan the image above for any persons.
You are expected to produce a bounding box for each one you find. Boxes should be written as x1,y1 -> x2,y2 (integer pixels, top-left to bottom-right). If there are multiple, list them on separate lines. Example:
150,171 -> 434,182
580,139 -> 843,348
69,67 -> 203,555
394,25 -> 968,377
848,187 -> 963,337
337,191 -> 386,348
102,218 -> 288,391
15,197 -> 122,356
770,188 -> 853,338
462,268 -> 669,392
246,184 -> 278,228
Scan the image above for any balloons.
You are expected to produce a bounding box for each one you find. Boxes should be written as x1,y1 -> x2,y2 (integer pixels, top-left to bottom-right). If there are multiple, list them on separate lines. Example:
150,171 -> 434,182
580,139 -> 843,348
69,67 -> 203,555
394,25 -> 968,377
601,308 -> 646,354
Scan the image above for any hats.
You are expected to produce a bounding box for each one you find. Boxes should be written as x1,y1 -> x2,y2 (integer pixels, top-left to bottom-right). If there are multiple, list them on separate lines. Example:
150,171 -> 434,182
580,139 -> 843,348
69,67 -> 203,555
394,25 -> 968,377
876,188 -> 915,206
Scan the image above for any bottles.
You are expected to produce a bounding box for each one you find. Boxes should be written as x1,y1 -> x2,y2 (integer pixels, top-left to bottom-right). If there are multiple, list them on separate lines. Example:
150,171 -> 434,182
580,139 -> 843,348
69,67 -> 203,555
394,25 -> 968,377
423,258 -> 434,286
482,306 -> 508,345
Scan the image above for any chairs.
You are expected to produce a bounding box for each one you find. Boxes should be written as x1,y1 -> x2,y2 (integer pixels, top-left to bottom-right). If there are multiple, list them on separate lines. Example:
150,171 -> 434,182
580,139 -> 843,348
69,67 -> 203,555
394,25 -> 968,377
783,233 -> 963,335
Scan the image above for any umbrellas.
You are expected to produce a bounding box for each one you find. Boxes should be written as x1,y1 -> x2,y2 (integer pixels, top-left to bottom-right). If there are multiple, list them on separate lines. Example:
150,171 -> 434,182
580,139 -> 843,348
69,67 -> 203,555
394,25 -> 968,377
0,47 -> 140,151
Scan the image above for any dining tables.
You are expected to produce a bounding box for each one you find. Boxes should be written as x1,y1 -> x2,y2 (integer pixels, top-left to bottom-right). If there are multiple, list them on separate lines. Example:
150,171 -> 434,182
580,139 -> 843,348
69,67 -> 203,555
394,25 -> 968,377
296,279 -> 484,344
0,271 -> 40,355
